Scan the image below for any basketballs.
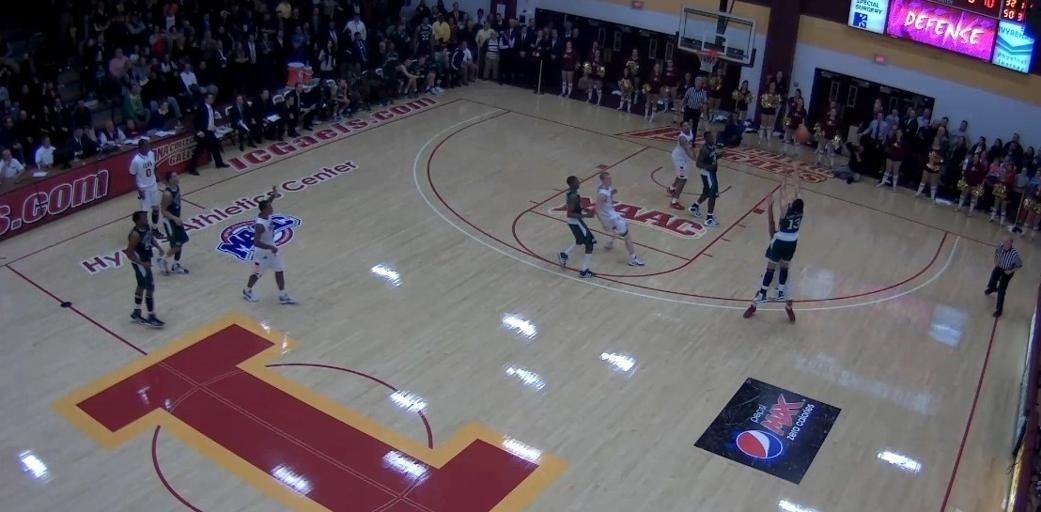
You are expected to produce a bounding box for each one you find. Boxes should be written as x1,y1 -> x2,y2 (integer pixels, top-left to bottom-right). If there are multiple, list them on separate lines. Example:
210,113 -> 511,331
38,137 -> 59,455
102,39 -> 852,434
793,124 -> 810,142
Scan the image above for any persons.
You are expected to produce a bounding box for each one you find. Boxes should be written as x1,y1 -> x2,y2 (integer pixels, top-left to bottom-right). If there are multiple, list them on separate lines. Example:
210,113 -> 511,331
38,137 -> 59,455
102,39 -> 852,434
666,121 -> 727,227
755,161 -> 804,303
594,172 -> 644,268
985,236 -> 1023,318
126,212 -> 164,327
241,185 -> 299,304
743,194 -> 796,323
1,0 -> 560,226
557,175 -> 597,278
779,89 -> 1041,239
157,169 -> 190,273
561,21 -> 789,147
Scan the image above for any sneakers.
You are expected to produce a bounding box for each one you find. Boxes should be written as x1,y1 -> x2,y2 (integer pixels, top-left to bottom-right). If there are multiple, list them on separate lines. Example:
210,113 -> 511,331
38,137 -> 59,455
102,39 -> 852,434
132,312 -> 166,327
776,290 -> 784,299
743,305 -> 756,318
689,207 -> 702,217
753,291 -> 766,304
627,255 -> 646,265
558,251 -> 568,270
579,269 -> 596,277
785,306 -> 795,321
279,295 -> 297,303
172,263 -> 188,273
242,287 -> 259,302
604,241 -> 613,248
667,187 -> 676,193
704,219 -> 718,227
670,202 -> 684,210
157,256 -> 171,273
151,229 -> 168,241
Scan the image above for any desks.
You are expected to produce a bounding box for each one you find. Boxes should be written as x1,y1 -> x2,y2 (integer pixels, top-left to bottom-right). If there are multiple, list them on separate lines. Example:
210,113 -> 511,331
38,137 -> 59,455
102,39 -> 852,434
1,120 -> 210,242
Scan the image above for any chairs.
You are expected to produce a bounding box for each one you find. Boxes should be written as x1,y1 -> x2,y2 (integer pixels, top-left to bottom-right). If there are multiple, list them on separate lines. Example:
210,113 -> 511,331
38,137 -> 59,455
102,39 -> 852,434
205,51 -> 453,154
